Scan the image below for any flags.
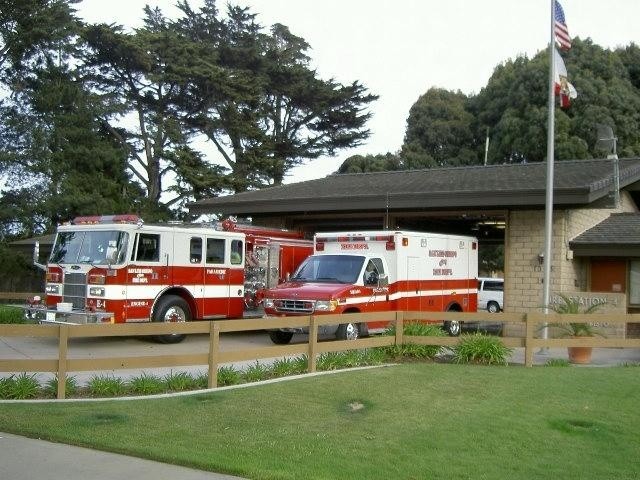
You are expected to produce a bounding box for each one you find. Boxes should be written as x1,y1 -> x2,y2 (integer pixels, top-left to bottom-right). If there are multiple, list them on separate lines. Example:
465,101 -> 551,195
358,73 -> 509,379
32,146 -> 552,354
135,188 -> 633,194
554,0 -> 571,49
553,46 -> 578,107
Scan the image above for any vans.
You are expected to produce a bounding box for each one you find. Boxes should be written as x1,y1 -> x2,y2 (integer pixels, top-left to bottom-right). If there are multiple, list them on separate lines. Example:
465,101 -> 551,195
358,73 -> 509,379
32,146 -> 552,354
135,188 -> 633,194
477,276 -> 505,314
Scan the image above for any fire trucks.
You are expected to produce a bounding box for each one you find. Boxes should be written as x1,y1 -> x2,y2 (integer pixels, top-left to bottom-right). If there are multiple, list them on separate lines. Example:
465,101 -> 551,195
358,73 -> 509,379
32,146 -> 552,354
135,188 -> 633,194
28,215 -> 316,344
261,231 -> 481,343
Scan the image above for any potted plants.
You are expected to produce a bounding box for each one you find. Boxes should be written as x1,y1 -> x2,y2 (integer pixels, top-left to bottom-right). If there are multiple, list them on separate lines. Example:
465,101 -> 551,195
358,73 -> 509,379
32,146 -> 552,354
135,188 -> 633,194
535,291 -> 609,364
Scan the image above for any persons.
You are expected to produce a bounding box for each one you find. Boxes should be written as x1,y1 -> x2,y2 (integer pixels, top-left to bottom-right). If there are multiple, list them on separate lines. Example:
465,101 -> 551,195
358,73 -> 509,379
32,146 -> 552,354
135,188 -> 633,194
363,262 -> 378,284
